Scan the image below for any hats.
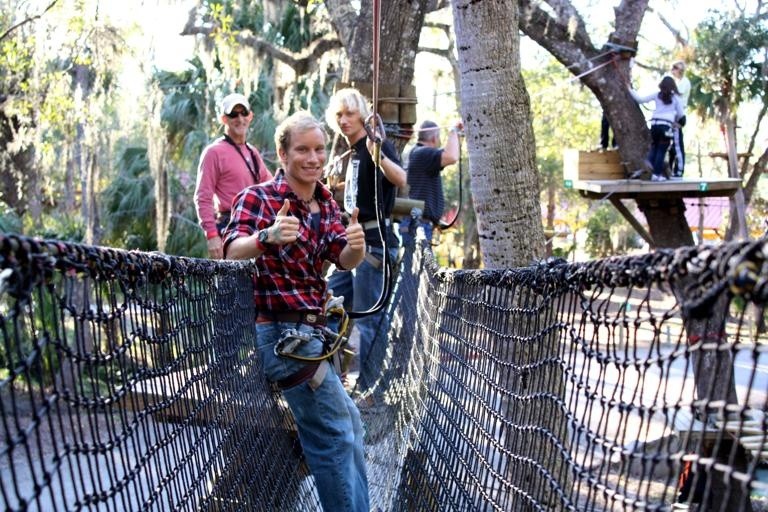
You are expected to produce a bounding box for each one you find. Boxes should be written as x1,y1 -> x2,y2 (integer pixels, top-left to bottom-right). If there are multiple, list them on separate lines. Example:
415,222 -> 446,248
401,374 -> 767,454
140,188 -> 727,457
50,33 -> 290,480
219,93 -> 250,114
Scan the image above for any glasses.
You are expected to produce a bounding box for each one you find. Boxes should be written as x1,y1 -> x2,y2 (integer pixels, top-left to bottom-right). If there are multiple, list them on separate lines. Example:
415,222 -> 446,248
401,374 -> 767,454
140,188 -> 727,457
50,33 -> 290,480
228,110 -> 247,118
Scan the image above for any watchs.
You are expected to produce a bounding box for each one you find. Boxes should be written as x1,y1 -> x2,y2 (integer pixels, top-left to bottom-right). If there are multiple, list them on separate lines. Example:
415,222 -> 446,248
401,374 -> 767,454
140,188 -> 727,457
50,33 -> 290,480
256,228 -> 270,246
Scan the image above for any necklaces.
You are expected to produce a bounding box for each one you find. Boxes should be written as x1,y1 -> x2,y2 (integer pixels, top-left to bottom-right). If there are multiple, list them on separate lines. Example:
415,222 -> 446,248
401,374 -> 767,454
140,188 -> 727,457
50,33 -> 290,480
234,146 -> 253,170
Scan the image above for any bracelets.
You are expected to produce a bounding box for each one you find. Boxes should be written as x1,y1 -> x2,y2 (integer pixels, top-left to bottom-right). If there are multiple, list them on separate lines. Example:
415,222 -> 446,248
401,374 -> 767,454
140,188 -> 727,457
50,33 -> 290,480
447,125 -> 460,133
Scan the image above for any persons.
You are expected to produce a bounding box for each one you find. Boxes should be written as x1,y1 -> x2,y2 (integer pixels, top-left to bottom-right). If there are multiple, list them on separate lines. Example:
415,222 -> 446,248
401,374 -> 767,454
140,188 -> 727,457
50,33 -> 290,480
664,57 -> 691,180
630,75 -> 683,183
409,119 -> 463,252
317,88 -> 406,395
598,107 -> 619,155
192,92 -> 274,262
221,109 -> 371,512
